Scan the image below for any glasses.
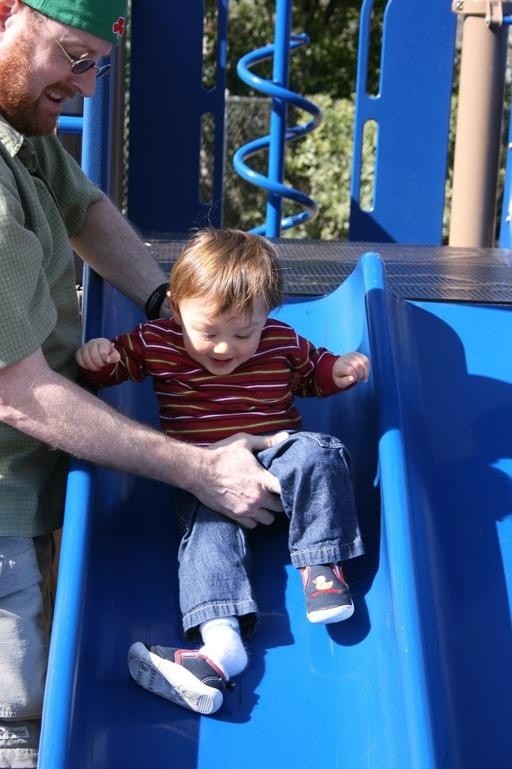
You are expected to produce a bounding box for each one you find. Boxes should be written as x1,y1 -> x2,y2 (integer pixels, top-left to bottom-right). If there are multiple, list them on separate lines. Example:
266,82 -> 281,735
35,12 -> 114,82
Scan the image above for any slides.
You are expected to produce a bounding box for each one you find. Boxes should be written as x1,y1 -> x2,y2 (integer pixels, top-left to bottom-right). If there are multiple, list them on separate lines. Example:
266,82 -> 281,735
37,250 -> 511,769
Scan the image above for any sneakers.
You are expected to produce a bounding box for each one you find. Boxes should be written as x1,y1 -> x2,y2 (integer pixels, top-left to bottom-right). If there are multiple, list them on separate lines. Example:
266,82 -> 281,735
125,639 -> 235,719
298,562 -> 356,628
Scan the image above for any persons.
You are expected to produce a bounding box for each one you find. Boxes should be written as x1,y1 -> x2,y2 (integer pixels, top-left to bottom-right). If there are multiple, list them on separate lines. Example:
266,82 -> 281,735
0,0 -> 288,769
76,228 -> 370,677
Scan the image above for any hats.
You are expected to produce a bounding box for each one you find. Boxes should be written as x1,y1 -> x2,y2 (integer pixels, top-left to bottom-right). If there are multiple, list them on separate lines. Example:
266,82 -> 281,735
19,0 -> 129,48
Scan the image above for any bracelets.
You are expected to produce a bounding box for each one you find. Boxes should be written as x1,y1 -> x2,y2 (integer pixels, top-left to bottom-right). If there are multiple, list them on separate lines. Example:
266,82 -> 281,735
145,282 -> 171,320
